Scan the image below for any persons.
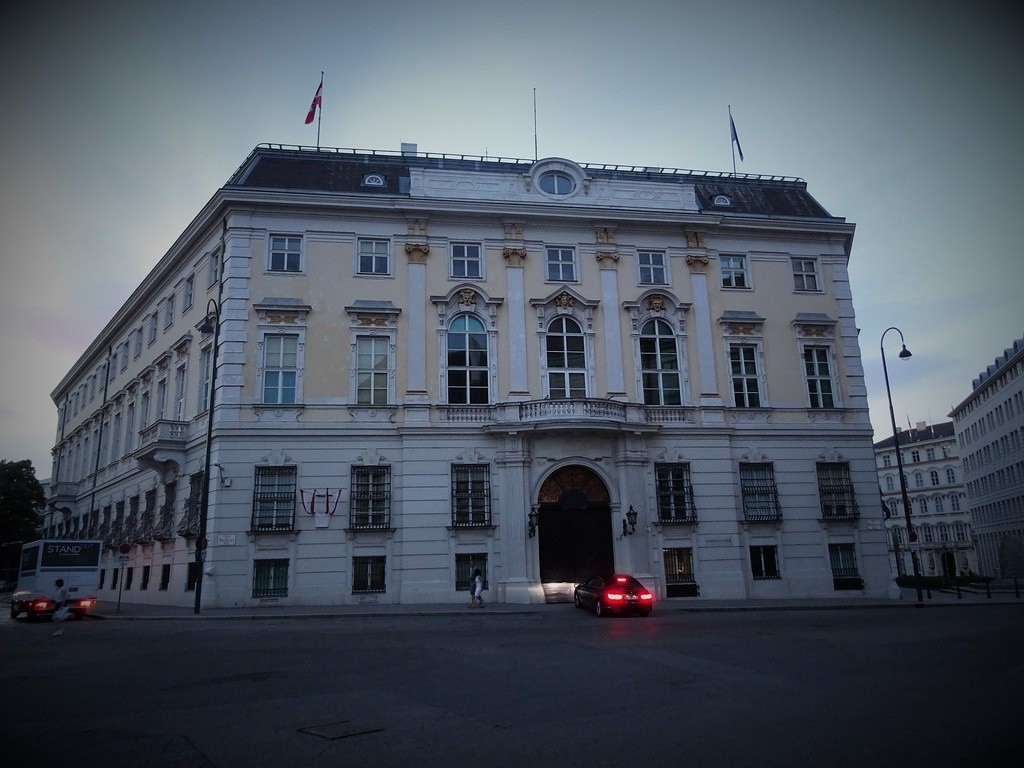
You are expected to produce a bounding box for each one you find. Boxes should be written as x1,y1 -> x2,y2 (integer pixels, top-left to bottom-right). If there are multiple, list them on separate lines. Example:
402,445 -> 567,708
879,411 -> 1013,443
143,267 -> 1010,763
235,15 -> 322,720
467,558 -> 484,608
51,579 -> 75,637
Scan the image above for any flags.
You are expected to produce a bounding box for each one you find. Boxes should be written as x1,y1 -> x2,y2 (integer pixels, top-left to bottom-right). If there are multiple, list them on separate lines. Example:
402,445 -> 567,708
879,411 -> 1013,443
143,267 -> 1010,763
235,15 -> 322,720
729,113 -> 744,162
304,79 -> 322,125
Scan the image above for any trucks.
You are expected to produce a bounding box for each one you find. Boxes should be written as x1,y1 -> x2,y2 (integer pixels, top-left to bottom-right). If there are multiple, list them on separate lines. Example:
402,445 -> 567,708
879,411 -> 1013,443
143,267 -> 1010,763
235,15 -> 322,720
11,539 -> 105,622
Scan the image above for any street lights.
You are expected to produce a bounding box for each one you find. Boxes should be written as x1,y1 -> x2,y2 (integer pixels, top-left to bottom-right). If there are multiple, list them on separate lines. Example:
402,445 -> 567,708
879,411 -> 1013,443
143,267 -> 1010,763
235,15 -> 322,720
193,298 -> 220,615
881,326 -> 925,602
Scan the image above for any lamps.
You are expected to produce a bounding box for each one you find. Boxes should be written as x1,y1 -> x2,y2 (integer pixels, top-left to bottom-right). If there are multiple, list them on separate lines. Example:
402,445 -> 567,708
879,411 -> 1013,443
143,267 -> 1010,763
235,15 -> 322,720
528,506 -> 539,538
623,505 -> 637,537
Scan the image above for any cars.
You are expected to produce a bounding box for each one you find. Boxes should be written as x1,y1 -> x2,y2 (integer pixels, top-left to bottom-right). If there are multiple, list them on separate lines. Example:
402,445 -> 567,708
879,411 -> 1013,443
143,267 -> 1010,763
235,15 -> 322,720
574,574 -> 653,618
